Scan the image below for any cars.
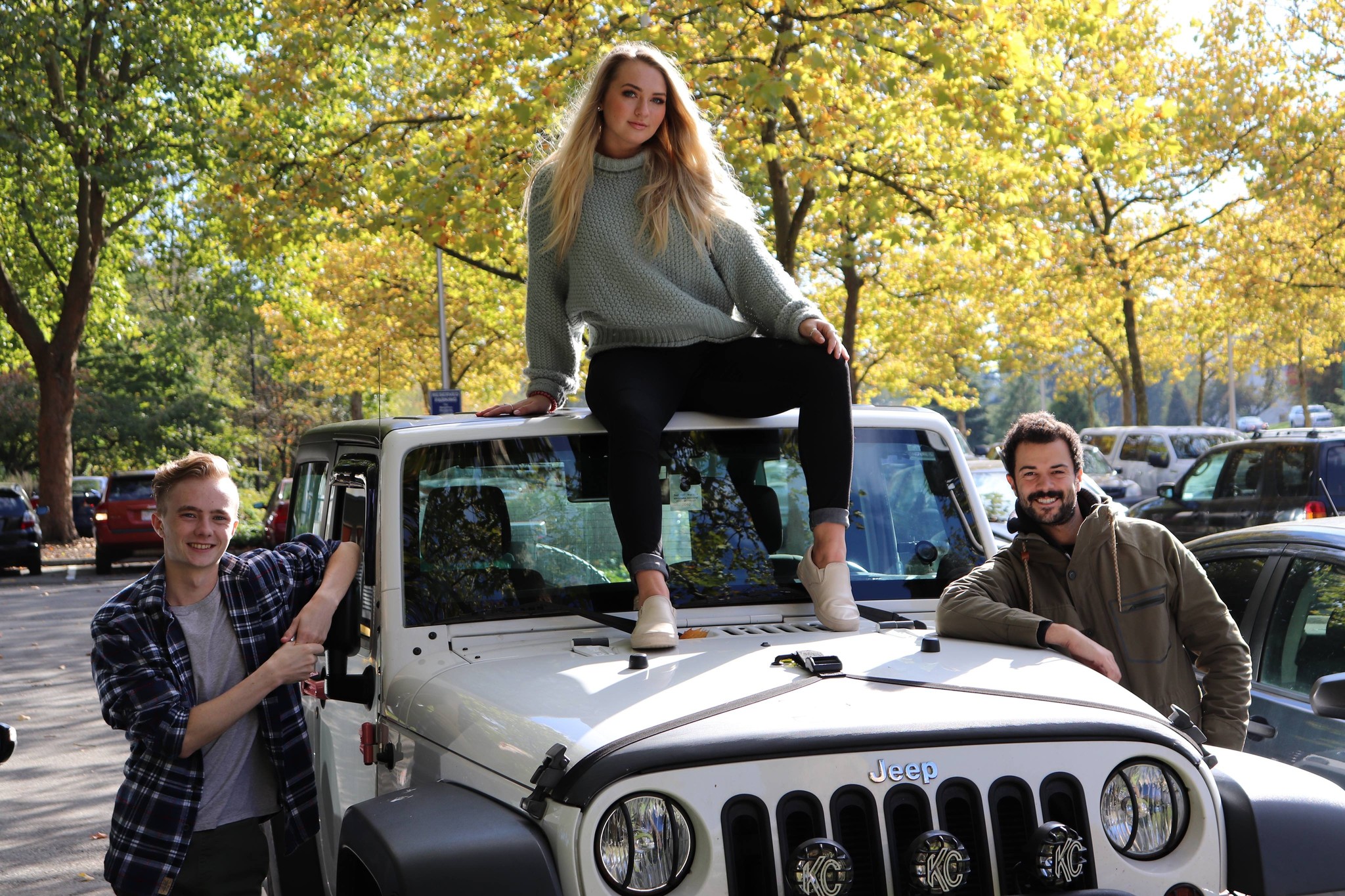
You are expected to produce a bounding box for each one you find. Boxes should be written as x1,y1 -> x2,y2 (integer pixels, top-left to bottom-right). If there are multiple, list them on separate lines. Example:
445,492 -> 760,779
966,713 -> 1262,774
1236,416 -> 1268,433
253,478 -> 294,548
1127,428 -> 1345,789
887,442 -> 1141,573
72,476 -> 108,538
0,482 -> 50,574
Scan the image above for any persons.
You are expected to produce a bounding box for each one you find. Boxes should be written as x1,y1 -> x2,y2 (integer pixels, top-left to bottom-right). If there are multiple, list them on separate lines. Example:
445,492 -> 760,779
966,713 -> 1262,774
476,40 -> 860,649
937,411 -> 1253,752
90,450 -> 361,896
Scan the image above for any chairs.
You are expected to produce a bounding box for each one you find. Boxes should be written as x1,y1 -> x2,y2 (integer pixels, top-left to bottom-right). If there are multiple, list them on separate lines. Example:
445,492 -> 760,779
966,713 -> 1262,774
670,483 -> 806,594
1245,462 -> 1260,493
421,486 -> 543,602
1294,602 -> 1345,693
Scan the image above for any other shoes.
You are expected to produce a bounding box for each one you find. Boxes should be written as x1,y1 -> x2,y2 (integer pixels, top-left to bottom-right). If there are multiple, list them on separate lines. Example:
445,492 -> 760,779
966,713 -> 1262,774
631,596 -> 679,649
797,546 -> 860,630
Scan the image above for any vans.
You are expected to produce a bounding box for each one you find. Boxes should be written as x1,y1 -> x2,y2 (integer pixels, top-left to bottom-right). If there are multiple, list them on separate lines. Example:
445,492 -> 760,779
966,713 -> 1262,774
1079,425 -> 1250,500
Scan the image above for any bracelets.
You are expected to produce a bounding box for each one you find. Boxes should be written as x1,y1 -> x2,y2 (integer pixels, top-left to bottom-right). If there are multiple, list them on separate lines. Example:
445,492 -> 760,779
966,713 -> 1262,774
530,391 -> 557,412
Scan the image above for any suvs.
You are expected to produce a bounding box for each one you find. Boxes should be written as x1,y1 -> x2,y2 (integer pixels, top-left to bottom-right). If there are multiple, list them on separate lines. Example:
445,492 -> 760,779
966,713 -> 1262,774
1289,404 -> 1334,427
286,402 -> 1345,896
94,469 -> 164,575
